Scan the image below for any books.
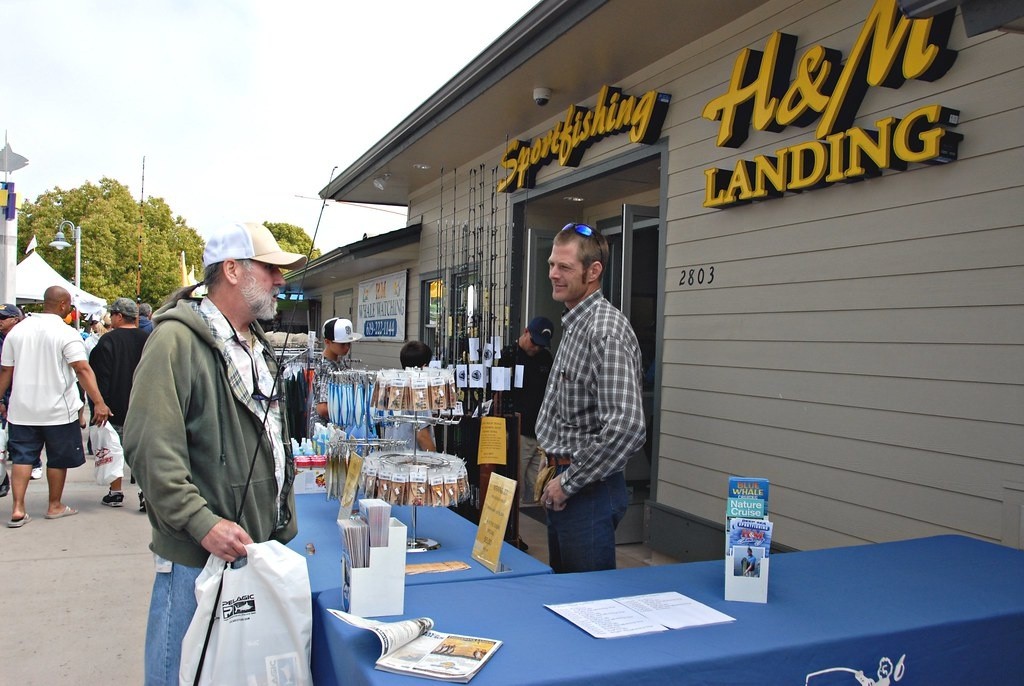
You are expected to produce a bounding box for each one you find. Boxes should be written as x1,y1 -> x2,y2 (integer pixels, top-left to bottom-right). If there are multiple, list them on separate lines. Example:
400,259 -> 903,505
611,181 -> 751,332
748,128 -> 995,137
327,608 -> 503,685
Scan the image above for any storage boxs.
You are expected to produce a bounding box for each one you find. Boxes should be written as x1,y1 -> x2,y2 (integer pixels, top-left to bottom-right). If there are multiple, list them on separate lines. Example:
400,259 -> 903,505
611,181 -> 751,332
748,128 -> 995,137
293,470 -> 328,494
725,553 -> 770,604
341,517 -> 407,618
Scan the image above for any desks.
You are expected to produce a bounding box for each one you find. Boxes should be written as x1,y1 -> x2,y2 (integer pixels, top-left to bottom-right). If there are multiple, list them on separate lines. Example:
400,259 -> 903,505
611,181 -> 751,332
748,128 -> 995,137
311,533 -> 1024,686
283,491 -> 556,612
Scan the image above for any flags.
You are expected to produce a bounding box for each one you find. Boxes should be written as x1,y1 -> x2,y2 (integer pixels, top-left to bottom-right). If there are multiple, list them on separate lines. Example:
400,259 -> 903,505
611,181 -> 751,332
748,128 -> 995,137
26,238 -> 37,254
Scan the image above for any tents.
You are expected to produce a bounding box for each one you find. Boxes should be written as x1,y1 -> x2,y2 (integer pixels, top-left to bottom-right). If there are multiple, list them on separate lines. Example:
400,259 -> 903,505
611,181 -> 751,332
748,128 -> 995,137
16,252 -> 107,329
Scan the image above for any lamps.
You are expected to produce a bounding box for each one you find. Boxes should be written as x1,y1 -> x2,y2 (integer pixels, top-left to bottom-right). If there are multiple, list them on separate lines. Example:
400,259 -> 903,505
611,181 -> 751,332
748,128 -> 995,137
373,172 -> 392,191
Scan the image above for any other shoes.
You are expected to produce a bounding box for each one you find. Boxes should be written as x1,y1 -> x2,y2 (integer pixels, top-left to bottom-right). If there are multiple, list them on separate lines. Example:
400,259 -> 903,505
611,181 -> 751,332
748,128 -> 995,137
0,473 -> 10,496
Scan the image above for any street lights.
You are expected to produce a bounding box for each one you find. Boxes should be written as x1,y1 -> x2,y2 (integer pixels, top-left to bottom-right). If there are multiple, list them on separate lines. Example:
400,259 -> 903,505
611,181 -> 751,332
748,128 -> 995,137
48,220 -> 82,332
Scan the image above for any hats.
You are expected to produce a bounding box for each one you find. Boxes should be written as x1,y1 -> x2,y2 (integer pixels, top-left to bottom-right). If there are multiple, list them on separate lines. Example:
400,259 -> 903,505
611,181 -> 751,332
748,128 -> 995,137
0,304 -> 23,322
322,316 -> 363,344
202,219 -> 308,272
105,298 -> 139,318
528,317 -> 554,348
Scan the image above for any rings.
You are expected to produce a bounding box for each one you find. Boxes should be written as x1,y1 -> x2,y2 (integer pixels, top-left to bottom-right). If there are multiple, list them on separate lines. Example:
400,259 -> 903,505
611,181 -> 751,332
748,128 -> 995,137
546,499 -> 552,507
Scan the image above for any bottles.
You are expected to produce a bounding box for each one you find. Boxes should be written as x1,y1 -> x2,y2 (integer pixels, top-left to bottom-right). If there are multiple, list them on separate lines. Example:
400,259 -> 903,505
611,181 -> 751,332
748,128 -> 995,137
291,422 -> 329,470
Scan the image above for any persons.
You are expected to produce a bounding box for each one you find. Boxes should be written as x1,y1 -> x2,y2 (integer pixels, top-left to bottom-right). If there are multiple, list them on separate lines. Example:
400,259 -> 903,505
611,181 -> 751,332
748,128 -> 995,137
0,304 -> 43,497
309,317 -> 362,438
534,223 -> 646,573
123,220 -> 308,686
81,298 -> 154,511
495,317 -> 554,503
379,341 -> 436,452
744,548 -> 756,577
0,286 -> 114,528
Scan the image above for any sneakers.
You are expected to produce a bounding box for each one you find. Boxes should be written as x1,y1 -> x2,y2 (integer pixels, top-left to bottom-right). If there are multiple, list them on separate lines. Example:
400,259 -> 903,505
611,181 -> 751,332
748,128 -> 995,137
102,489 -> 125,506
28,466 -> 42,480
136,492 -> 148,512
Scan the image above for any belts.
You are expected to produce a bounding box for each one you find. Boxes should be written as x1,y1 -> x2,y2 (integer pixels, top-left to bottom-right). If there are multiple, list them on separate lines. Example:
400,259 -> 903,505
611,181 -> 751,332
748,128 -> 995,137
545,455 -> 572,467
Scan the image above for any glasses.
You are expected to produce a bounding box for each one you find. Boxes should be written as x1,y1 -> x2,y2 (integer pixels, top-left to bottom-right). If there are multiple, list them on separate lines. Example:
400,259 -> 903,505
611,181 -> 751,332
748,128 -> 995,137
529,335 -> 548,348
109,309 -> 122,316
563,224 -> 603,265
0,314 -> 14,320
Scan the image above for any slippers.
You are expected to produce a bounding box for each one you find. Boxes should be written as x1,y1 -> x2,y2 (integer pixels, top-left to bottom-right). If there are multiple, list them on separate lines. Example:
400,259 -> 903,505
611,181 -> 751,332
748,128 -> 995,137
45,505 -> 78,518
7,512 -> 32,527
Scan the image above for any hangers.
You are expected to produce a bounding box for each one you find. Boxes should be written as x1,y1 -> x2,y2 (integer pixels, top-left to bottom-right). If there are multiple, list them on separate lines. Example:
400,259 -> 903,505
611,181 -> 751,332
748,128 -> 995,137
281,351 -> 303,382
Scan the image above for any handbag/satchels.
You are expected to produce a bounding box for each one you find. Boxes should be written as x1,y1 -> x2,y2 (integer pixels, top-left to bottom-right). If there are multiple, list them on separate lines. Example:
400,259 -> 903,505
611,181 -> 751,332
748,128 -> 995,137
89,421 -> 124,485
179,540 -> 314,686
